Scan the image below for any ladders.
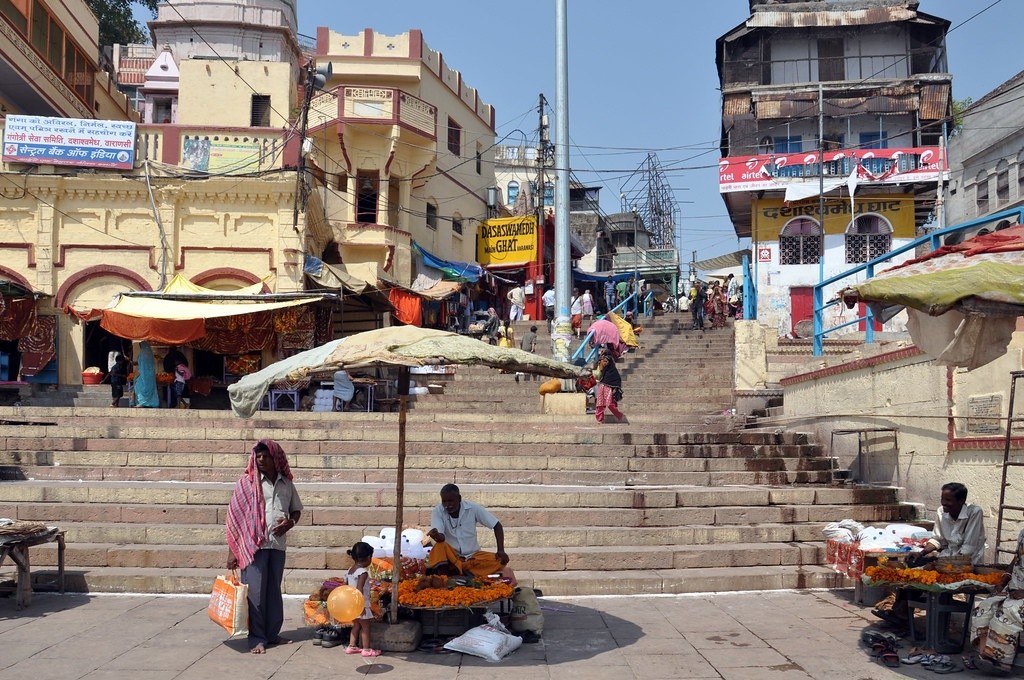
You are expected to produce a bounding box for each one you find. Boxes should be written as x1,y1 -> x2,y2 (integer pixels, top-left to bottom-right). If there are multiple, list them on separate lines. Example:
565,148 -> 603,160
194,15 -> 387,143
993,371 -> 1024,567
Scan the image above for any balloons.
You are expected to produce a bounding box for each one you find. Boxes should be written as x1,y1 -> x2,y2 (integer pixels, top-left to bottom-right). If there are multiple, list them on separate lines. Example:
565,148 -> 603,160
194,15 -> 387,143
327,585 -> 365,622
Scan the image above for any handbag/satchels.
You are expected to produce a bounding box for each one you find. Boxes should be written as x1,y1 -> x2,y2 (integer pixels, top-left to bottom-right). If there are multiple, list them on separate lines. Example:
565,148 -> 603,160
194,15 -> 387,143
511,587 -> 544,636
207,567 -> 249,636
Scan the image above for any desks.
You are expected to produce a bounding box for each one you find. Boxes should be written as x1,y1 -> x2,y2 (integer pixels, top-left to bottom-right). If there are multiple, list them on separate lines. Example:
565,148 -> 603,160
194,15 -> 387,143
826,538 -> 910,604
0,527 -> 69,611
375,380 -> 394,399
321,381 -> 374,413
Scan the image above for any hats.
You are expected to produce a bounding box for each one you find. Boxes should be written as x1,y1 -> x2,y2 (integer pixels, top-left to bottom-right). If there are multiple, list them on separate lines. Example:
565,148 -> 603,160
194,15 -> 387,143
573,288 -> 578,293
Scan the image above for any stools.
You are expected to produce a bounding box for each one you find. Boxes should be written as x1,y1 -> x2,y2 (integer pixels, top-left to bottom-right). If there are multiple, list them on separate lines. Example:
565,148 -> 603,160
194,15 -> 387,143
258,390 -> 300,412
908,586 -> 975,653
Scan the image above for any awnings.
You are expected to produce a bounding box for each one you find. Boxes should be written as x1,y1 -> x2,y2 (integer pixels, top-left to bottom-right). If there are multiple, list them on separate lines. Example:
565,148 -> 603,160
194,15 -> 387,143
303,240 -> 517,314
828,224 -> 1024,371
68,274 -> 339,353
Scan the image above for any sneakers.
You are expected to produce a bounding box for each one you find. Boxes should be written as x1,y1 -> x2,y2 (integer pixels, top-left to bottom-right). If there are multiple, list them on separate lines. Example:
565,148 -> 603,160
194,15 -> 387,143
313,627 -> 329,644
322,630 -> 346,647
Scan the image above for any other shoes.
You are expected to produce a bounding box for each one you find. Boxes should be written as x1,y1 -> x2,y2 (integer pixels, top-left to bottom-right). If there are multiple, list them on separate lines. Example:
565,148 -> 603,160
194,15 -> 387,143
346,646 -> 363,654
361,648 -> 381,656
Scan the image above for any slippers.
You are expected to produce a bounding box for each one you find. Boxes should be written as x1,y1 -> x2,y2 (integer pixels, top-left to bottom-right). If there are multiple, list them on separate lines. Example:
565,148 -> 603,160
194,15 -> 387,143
964,655 -> 985,669
865,631 -> 903,667
901,654 -> 964,673
871,608 -> 908,628
974,658 -> 1011,676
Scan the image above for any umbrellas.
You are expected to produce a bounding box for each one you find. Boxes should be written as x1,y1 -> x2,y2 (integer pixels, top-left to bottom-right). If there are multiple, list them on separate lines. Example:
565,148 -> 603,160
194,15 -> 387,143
227,325 -> 592,624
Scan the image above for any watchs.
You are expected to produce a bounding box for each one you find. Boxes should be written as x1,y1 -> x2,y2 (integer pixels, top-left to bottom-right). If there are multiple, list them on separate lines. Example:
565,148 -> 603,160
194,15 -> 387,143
290,519 -> 295,526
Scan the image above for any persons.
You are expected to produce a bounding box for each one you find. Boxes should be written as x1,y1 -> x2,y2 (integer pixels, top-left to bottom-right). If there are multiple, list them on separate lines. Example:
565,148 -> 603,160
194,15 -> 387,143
871,482 -> 985,624
573,348 -> 625,425
542,282 -> 594,340
227,440 -> 301,654
425,484 -> 510,577
110,355 -> 127,408
661,273 -> 743,330
124,341 -> 160,408
603,275 -> 648,336
961,511 -> 1024,680
507,284 -> 526,321
332,369 -> 354,412
483,307 -> 537,381
173,355 -> 191,408
343,541 -> 381,657
